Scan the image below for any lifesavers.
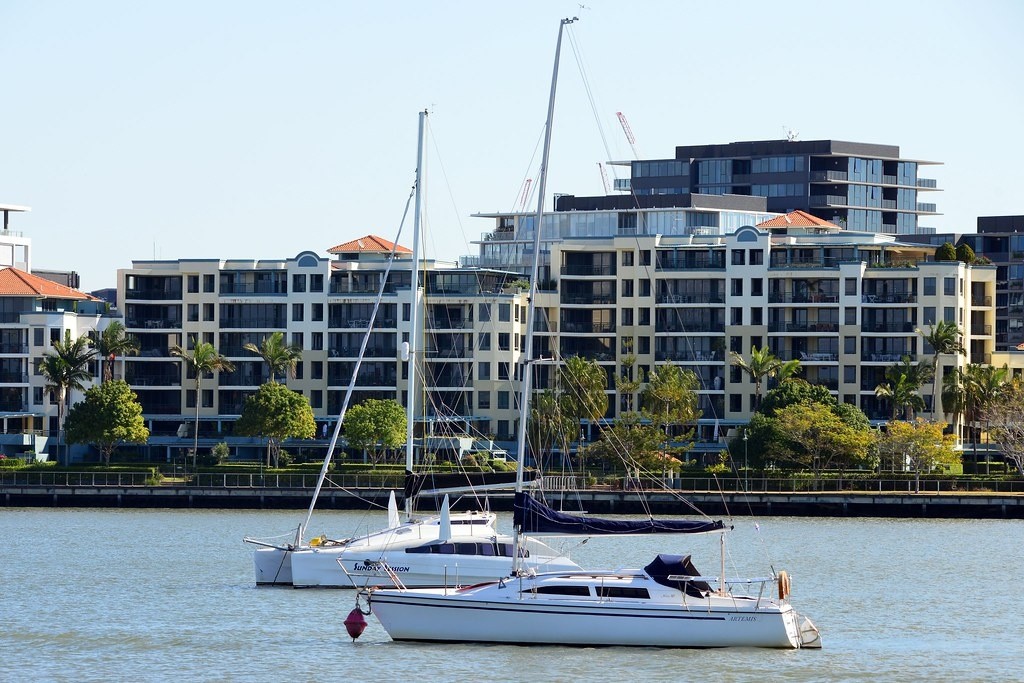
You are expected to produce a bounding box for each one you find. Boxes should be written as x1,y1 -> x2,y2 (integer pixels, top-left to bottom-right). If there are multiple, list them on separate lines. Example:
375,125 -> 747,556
778,571 -> 790,599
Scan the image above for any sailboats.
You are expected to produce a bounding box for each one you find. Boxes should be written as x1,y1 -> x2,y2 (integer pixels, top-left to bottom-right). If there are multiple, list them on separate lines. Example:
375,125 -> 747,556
244,101 -> 581,589
336,9 -> 827,648
